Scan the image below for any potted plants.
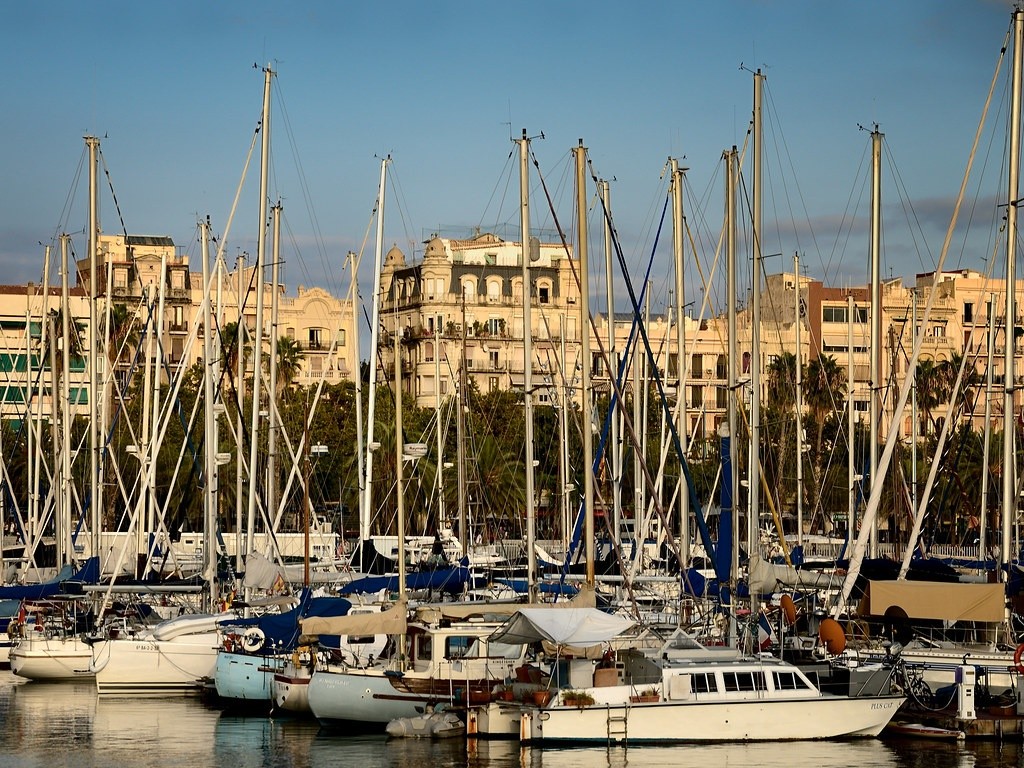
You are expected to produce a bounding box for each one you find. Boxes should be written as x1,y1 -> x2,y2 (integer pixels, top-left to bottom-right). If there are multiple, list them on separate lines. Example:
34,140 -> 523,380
497,684 -> 661,714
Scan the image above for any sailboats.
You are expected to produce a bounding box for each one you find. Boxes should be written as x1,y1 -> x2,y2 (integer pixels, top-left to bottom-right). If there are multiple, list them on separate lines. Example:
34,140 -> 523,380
3,0 -> 1023,742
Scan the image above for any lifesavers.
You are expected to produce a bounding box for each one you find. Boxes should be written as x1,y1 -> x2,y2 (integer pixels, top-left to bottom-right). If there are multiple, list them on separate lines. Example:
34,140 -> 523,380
241,626 -> 266,653
1013,644 -> 1024,676
291,646 -> 318,668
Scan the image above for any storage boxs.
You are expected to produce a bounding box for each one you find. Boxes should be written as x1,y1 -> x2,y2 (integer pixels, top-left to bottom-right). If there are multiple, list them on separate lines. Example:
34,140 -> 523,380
594,668 -> 618,687
